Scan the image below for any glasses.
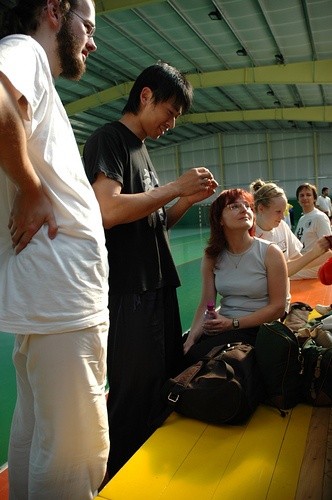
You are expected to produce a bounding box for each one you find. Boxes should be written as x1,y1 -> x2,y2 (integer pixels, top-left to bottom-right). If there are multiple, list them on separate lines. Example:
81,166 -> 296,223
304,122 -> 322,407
69,8 -> 96,38
225,202 -> 255,210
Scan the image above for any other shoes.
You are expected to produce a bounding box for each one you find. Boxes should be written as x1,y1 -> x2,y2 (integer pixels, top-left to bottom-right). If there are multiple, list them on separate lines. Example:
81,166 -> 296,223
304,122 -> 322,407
315,304 -> 332,315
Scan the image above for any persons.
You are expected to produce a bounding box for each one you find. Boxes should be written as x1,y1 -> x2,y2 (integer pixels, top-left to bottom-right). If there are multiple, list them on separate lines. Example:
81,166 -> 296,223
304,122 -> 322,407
184,189 -> 286,370
0,0 -> 110,500
249,180 -> 329,277
84,62 -> 218,481
289,183 -> 332,280
315,187 -> 332,221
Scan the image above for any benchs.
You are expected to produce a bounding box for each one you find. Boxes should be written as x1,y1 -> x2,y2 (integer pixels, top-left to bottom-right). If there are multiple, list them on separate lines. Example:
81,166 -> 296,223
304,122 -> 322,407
91,279 -> 332,500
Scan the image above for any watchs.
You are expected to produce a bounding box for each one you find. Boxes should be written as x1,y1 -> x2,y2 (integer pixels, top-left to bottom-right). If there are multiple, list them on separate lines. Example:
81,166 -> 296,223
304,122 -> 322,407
233,318 -> 239,329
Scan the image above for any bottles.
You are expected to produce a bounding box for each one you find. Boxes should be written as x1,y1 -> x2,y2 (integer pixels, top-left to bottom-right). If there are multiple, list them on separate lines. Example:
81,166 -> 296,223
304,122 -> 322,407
203,301 -> 216,335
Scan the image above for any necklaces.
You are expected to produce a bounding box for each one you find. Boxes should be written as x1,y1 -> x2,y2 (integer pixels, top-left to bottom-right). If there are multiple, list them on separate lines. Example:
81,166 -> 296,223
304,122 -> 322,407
229,253 -> 242,269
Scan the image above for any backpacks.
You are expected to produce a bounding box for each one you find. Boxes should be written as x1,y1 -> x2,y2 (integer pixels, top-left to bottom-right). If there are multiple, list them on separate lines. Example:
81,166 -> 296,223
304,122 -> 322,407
168,343 -> 262,426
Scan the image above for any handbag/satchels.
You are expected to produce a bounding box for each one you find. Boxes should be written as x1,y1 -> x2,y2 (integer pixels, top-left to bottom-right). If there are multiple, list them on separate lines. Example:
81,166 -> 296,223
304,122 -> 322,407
254,321 -> 299,409
313,316 -> 332,330
285,302 -> 313,329
300,337 -> 332,409
